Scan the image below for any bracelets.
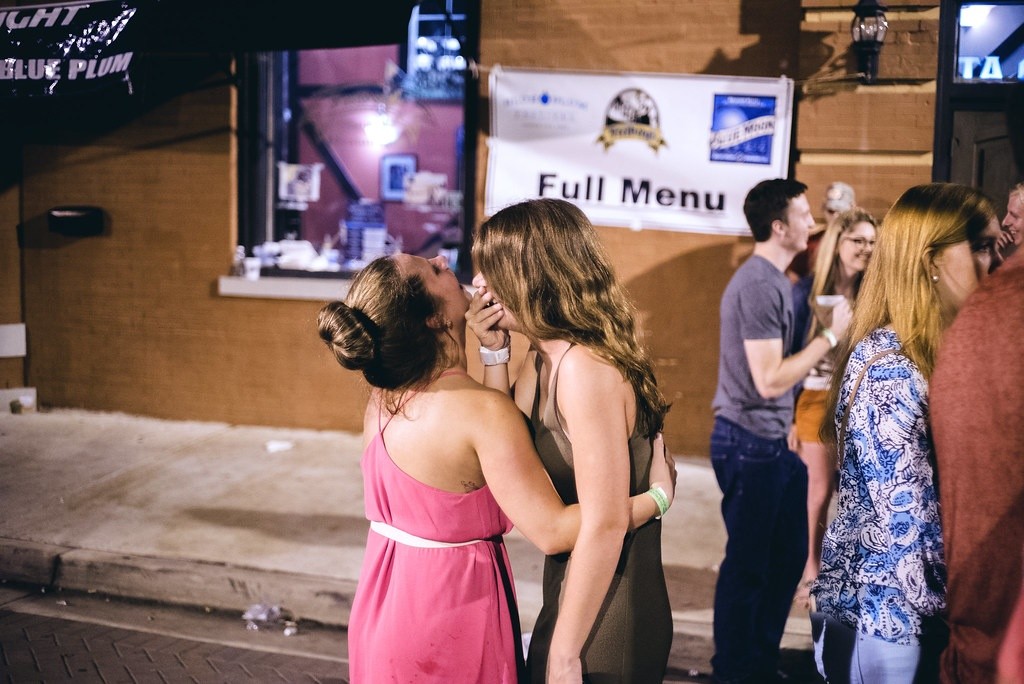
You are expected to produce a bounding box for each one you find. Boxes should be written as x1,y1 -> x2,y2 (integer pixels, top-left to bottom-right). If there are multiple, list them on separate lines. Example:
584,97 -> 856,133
821,331 -> 837,348
478,345 -> 511,366
648,488 -> 669,520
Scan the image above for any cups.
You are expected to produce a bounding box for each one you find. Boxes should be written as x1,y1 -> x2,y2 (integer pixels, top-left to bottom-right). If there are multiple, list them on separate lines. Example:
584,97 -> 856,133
243,257 -> 261,279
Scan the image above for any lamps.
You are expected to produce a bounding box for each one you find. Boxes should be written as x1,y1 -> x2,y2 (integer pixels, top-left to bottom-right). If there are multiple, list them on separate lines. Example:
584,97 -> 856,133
849,0 -> 888,83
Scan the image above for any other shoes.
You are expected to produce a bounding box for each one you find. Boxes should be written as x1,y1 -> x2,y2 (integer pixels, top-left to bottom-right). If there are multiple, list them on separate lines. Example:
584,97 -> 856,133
775,668 -> 810,684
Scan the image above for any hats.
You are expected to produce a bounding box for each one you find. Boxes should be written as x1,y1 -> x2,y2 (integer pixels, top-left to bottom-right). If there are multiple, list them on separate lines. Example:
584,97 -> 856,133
826,181 -> 856,211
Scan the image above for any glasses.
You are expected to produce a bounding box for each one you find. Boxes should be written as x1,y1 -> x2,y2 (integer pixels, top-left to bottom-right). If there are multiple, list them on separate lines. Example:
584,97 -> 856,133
842,235 -> 875,248
824,207 -> 835,213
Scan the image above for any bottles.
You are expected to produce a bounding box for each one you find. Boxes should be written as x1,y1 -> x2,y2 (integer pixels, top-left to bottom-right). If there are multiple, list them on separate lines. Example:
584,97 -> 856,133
234,245 -> 245,277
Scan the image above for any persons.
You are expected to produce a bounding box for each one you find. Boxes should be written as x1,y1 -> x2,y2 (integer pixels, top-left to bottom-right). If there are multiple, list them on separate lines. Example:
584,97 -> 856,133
929,244 -> 1024,684
809,183 -> 1004,684
316,250 -> 677,684
788,208 -> 877,607
997,184 -> 1024,250
463,199 -> 673,684
785,249 -> 816,399
708,179 -> 855,684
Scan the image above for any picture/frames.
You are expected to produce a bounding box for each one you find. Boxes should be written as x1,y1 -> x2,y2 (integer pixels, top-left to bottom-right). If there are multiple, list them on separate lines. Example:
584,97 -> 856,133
378,153 -> 418,203
